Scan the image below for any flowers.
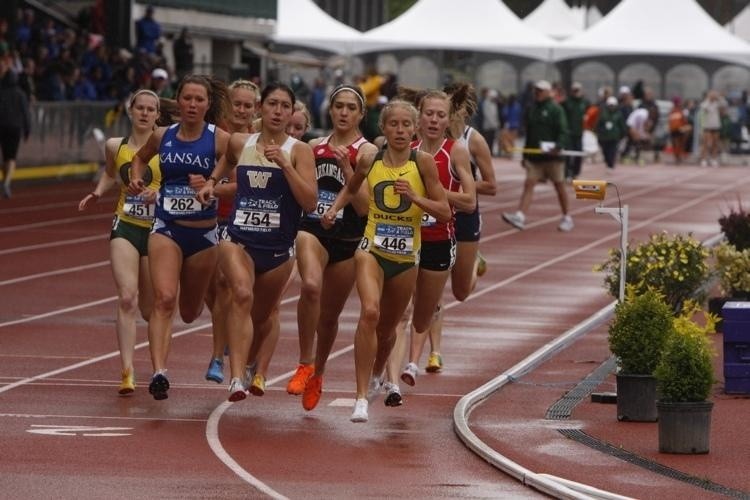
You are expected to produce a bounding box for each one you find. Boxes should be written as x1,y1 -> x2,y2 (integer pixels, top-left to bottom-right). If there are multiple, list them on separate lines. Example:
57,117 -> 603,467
595,230 -> 716,300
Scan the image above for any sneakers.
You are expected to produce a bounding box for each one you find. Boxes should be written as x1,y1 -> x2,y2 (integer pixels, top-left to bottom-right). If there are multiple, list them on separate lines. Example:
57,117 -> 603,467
148,371 -> 170,400
558,218 -> 573,231
205,352 -> 443,423
476,252 -> 487,276
117,367 -> 137,396
502,212 -> 524,230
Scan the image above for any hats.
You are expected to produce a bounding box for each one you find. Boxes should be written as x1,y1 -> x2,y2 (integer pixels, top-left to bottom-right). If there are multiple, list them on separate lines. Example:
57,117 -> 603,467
535,81 -> 631,105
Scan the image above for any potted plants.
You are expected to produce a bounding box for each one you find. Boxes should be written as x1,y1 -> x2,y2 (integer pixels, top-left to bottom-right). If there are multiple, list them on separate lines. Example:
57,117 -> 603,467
651,299 -> 722,455
609,283 -> 672,422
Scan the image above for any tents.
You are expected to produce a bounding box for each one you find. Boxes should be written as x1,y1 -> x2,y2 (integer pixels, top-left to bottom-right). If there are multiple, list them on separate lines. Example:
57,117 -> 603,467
246,0 -> 750,99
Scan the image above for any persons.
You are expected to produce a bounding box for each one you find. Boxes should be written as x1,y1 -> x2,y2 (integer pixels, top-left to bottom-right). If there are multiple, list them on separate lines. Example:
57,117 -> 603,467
229,101 -> 312,397
466,75 -> 596,233
420,81 -> 499,374
321,97 -> 454,423
201,78 -> 266,393
1,3 -> 194,200
372,89 -> 479,393
75,88 -> 173,396
593,78 -> 750,170
127,74 -> 221,402
194,81 -> 320,403
291,65 -> 417,139
286,85 -> 378,410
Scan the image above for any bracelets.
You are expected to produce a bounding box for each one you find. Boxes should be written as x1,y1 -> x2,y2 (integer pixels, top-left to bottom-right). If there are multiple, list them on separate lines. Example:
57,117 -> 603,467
88,192 -> 98,199
207,175 -> 217,184
